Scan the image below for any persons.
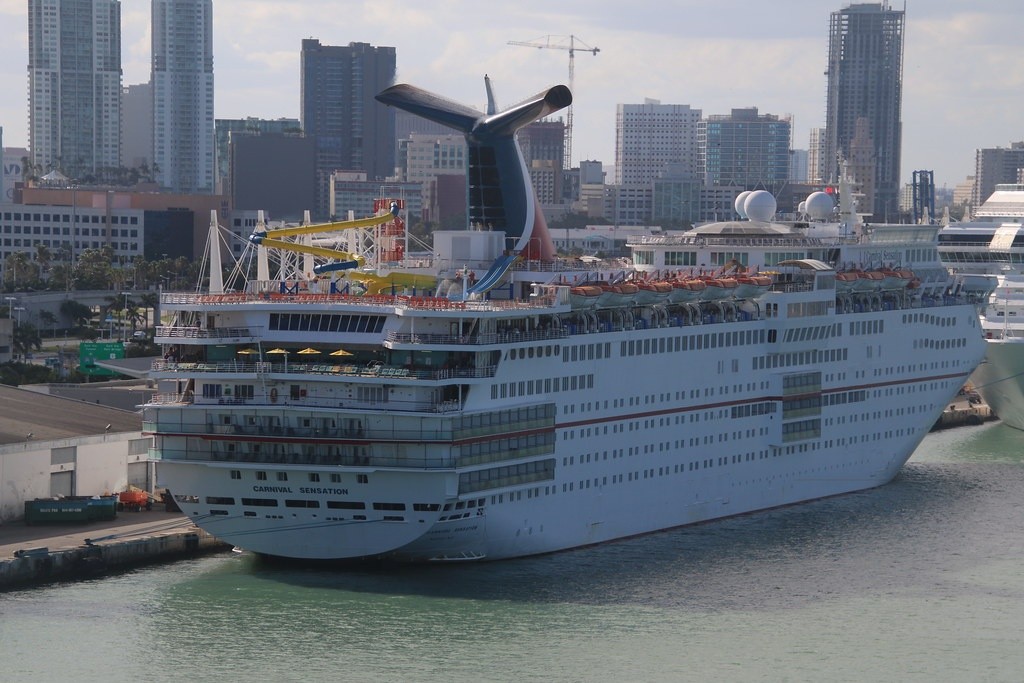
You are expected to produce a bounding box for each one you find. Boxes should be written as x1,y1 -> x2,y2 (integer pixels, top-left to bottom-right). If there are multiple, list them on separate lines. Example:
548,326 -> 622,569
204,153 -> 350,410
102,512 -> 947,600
313,277 -> 318,293
164,347 -> 202,363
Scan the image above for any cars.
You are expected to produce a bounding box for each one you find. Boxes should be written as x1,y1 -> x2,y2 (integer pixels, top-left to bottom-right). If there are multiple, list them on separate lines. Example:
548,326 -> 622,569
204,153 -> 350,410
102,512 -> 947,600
132,331 -> 146,340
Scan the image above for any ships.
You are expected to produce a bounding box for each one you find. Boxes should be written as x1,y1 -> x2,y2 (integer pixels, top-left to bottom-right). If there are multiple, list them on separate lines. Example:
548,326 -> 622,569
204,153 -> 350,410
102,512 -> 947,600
136,79 -> 1000,566
934,170 -> 1023,429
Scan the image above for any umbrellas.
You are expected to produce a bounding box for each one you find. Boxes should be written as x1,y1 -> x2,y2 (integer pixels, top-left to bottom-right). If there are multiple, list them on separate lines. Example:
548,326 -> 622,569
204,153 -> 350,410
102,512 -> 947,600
266,349 -> 290,362
297,348 -> 321,364
329,349 -> 354,365
237,348 -> 260,361
